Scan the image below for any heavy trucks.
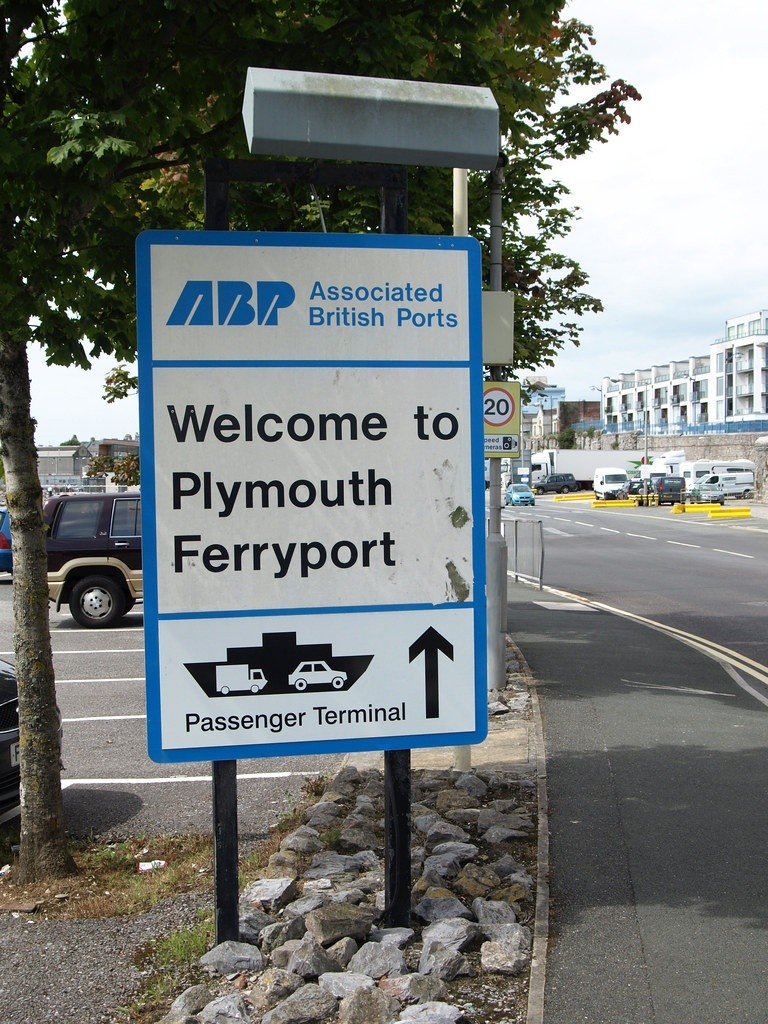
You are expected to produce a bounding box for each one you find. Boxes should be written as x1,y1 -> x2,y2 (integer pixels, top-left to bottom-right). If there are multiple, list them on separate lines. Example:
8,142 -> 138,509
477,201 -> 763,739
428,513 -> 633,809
517,450 -> 654,490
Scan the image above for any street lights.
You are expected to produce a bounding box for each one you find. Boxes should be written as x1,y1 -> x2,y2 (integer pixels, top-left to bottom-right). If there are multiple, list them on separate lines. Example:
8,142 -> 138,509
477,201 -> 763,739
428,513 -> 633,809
683,373 -> 696,429
610,378 -> 647,508
544,394 -> 552,434
725,351 -> 743,434
590,385 -> 602,433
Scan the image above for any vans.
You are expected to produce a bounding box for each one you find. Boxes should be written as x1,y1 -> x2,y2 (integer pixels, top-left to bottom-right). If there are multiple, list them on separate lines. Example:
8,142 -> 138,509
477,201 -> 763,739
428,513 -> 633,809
685,472 -> 755,502
593,468 -> 631,498
651,475 -> 685,504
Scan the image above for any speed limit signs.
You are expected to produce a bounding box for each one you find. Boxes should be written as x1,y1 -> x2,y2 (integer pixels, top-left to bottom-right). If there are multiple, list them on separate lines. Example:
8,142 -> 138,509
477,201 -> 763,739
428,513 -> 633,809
483,387 -> 514,427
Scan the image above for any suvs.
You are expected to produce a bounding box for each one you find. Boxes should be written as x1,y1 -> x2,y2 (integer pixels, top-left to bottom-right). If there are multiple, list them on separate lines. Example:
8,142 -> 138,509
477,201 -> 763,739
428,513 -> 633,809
616,479 -> 652,501
45,492 -> 144,626
532,473 -> 576,494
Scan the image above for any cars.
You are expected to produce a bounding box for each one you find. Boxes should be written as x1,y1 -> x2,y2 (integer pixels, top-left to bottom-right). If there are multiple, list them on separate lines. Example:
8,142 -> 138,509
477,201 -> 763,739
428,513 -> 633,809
0,657 -> 61,840
505,484 -> 536,507
1,512 -> 15,575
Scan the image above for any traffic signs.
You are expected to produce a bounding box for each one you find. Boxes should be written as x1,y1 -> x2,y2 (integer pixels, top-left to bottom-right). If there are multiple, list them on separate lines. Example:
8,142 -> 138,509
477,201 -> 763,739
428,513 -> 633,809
136,230 -> 488,762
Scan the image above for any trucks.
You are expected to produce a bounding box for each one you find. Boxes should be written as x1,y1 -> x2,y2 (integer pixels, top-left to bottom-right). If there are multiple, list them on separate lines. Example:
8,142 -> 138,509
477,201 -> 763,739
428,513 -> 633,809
485,459 -> 510,487
650,451 -> 687,477
